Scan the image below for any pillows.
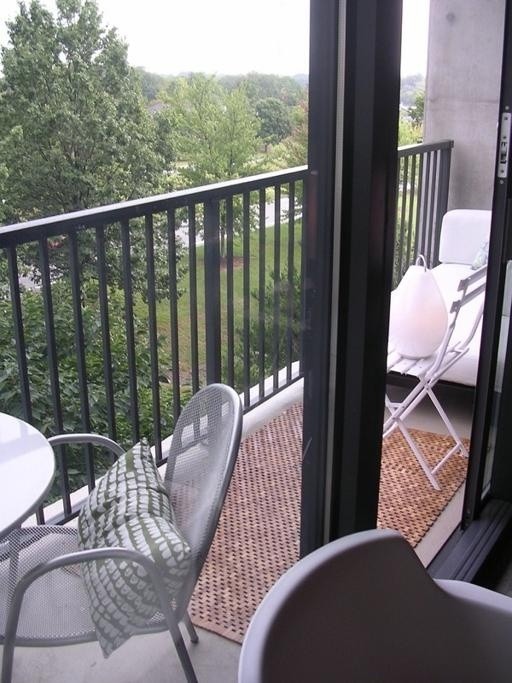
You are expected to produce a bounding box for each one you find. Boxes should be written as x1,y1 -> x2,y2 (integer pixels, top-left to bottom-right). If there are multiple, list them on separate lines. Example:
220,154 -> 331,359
76,436 -> 192,659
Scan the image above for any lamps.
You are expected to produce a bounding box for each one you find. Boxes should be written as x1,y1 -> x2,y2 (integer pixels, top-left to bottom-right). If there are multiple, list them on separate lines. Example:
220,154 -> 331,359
390,255 -> 448,359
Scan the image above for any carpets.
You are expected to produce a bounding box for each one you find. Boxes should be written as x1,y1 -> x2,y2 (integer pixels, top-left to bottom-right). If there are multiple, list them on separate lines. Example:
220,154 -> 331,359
62,399 -> 491,645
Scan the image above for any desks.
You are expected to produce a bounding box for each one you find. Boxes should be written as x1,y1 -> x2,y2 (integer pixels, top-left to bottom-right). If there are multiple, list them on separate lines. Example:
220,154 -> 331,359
0,412 -> 57,542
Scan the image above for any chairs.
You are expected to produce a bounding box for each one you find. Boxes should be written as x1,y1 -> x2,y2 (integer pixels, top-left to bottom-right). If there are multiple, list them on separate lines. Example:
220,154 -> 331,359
382,262 -> 489,492
238,528 -> 512,683
0,382 -> 242,683
419,209 -> 512,426
